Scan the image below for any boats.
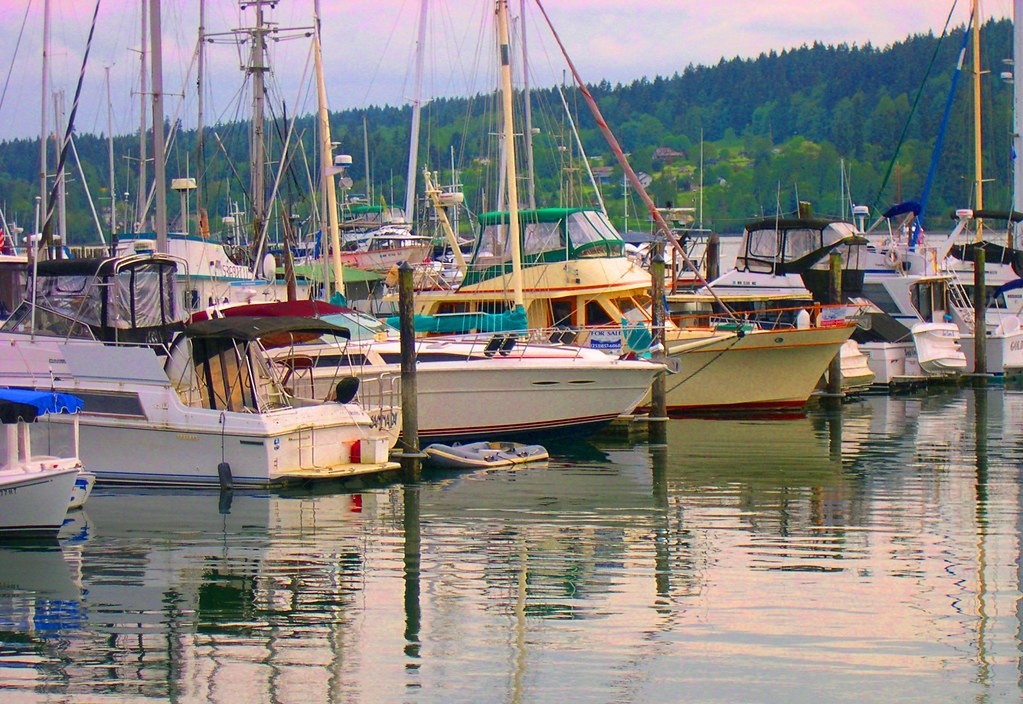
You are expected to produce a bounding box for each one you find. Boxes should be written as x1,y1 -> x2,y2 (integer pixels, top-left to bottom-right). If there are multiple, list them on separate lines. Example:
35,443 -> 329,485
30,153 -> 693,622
419,440 -> 551,469
0,387 -> 87,538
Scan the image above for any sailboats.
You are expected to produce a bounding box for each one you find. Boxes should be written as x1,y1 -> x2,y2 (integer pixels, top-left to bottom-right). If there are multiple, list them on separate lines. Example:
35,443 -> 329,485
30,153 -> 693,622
1,2 -> 1023,490
